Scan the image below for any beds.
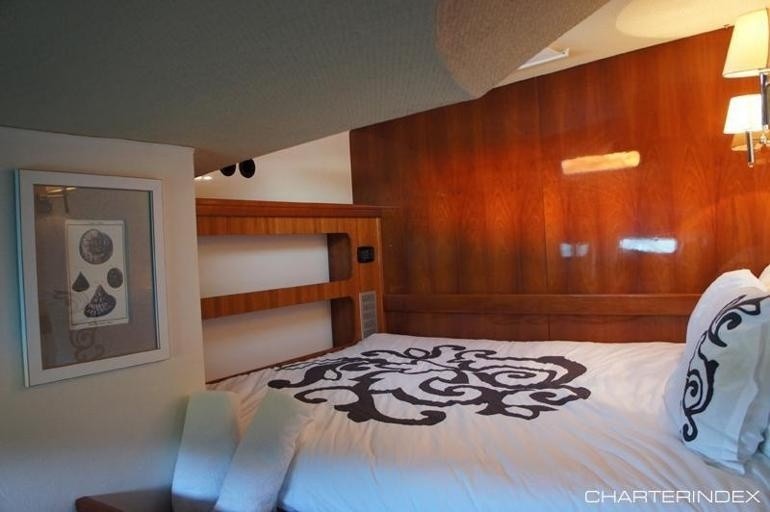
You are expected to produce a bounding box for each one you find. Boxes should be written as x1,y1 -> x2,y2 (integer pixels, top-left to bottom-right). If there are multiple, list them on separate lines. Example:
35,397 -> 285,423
194,260 -> 770,512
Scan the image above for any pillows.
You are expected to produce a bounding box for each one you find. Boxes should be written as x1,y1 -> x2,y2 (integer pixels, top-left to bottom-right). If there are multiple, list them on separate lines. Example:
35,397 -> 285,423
169,384 -> 238,511
212,386 -> 316,512
652,282 -> 770,475
682,262 -> 770,461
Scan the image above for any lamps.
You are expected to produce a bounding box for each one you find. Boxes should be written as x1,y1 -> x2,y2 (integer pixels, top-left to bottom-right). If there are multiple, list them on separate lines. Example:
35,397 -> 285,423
717,6 -> 770,144
730,135 -> 769,154
719,95 -> 768,169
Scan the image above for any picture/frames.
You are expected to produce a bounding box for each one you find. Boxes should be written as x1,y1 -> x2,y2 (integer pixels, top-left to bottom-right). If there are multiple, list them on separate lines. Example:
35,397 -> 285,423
10,166 -> 173,392
